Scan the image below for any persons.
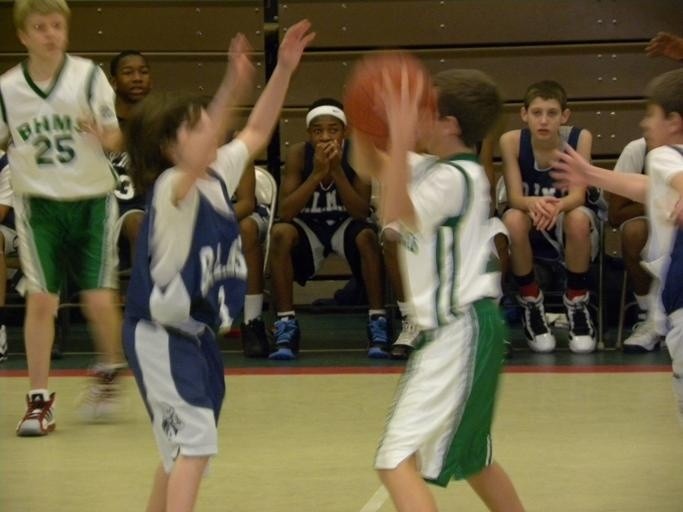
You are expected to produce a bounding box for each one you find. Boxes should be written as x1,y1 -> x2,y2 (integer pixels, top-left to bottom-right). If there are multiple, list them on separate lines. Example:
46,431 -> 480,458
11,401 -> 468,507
344,69 -> 524,511
122,20 -> 316,512
266,98 -> 394,363
498,81 -> 598,353
1,0 -> 155,436
549,28 -> 682,413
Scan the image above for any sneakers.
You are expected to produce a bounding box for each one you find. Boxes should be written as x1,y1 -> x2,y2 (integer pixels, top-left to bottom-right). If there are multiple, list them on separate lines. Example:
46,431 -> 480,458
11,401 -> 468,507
363,317 -> 389,357
516,290 -> 556,352
269,314 -> 300,359
16,388 -> 56,436
73,363 -> 131,418
624,308 -> 666,352
391,320 -> 422,358
240,317 -> 269,357
562,291 -> 596,352
0,325 -> 10,361
50,310 -> 70,357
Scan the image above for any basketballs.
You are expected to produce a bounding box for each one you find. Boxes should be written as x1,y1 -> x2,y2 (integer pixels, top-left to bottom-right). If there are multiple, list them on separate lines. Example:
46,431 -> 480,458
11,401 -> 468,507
346,54 -> 435,141
